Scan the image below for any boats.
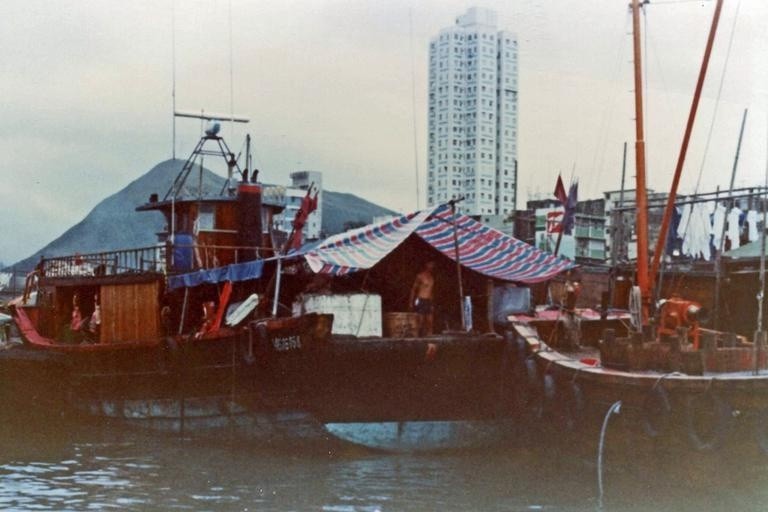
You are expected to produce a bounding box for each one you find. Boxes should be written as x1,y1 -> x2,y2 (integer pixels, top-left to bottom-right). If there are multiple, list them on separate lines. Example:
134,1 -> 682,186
8,106 -> 581,456
504,0 -> 768,490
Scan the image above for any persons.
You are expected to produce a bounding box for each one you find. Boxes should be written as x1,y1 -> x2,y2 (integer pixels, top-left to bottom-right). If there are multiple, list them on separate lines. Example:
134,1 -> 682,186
91,290 -> 101,344
71,293 -> 83,342
71,248 -> 86,268
404,256 -> 439,333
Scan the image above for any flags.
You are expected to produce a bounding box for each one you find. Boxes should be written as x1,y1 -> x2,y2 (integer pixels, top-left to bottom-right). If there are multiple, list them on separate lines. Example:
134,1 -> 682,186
545,173 -> 567,234
559,178 -> 588,236
